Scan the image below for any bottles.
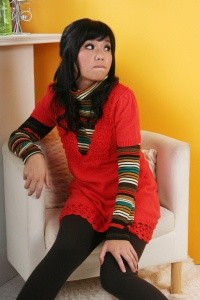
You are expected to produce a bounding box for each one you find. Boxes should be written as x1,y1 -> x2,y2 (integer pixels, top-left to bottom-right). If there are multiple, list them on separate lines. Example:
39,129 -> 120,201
0,0 -> 12,35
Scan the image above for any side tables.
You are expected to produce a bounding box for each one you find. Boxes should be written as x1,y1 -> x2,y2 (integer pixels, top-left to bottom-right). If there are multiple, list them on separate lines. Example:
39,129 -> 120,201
0,33 -> 63,147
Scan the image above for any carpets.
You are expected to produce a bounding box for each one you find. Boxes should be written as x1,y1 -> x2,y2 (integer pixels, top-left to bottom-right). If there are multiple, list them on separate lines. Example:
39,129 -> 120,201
0,256 -> 200,300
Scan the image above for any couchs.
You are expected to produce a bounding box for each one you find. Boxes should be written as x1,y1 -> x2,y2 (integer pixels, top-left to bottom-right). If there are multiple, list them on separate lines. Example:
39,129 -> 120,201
2,130 -> 190,294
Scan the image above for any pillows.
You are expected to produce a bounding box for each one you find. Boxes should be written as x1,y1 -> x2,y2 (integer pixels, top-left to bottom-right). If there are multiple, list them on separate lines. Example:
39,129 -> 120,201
45,139 -> 158,209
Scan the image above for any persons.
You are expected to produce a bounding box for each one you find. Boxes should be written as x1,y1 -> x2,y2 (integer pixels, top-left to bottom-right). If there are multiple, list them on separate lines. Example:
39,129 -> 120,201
8,19 -> 168,300
